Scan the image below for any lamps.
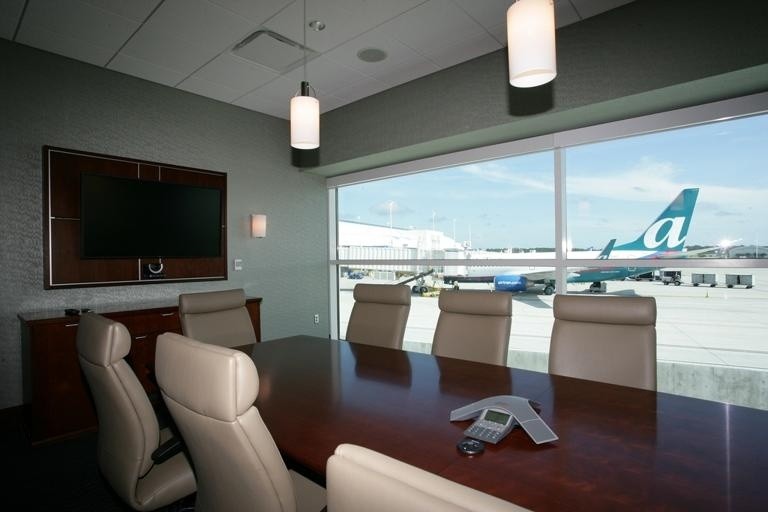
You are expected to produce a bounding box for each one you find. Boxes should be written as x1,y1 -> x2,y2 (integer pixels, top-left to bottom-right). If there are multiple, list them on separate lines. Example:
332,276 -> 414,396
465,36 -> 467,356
290,0 -> 320,149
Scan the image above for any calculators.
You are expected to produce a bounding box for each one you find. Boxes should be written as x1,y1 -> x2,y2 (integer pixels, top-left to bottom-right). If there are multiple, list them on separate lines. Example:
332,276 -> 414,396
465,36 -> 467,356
463,408 -> 514,445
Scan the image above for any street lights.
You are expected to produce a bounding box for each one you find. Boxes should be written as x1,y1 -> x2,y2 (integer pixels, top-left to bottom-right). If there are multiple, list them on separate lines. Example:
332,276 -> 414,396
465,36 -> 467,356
432,209 -> 436,233
386,198 -> 393,233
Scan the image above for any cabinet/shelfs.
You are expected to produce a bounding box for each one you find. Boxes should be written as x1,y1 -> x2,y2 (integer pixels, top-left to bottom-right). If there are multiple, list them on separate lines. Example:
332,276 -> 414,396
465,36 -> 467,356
17,295 -> 263,448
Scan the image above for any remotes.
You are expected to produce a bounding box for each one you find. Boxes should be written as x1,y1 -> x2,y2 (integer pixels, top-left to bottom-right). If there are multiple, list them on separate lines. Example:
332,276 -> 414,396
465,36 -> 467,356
65,309 -> 79,314
82,309 -> 95,313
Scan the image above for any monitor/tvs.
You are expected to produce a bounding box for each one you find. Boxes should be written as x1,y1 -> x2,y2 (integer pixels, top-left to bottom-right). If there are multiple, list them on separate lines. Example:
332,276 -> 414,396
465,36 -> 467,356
81,172 -> 221,259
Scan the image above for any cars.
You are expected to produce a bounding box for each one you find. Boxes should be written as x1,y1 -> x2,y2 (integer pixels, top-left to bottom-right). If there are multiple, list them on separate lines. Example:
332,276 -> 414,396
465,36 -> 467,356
348,272 -> 365,279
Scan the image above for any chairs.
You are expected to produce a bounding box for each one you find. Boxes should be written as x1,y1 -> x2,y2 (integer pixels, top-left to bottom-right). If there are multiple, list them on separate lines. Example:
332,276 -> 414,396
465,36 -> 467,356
153,333 -> 328,512
345,284 -> 411,349
507,0 -> 557,88
252,214 -> 266,238
325,443 -> 533,512
177,288 -> 257,347
430,289 -> 512,367
547,294 -> 657,392
76,312 -> 196,512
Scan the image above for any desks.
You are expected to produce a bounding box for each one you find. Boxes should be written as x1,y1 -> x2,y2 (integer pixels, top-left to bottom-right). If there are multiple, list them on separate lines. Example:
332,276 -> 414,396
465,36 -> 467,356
234,336 -> 768,512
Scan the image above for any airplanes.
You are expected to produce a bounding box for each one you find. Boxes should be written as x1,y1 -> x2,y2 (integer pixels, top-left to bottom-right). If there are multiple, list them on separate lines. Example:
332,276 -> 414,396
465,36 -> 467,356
339,186 -> 745,297
